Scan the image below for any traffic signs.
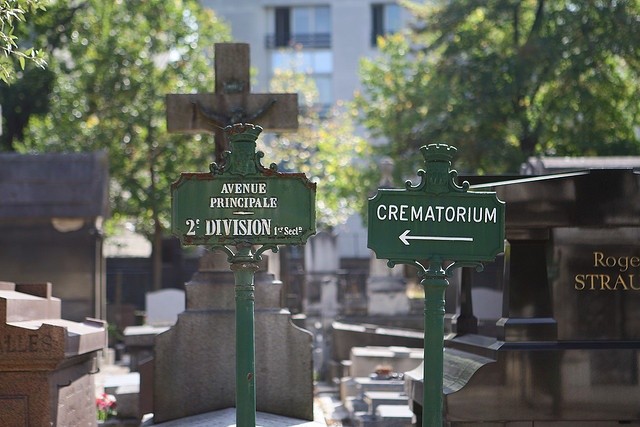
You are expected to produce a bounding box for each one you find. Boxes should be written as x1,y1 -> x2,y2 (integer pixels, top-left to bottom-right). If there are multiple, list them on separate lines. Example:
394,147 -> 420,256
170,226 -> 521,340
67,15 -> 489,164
368,189 -> 506,261
171,172 -> 316,245
